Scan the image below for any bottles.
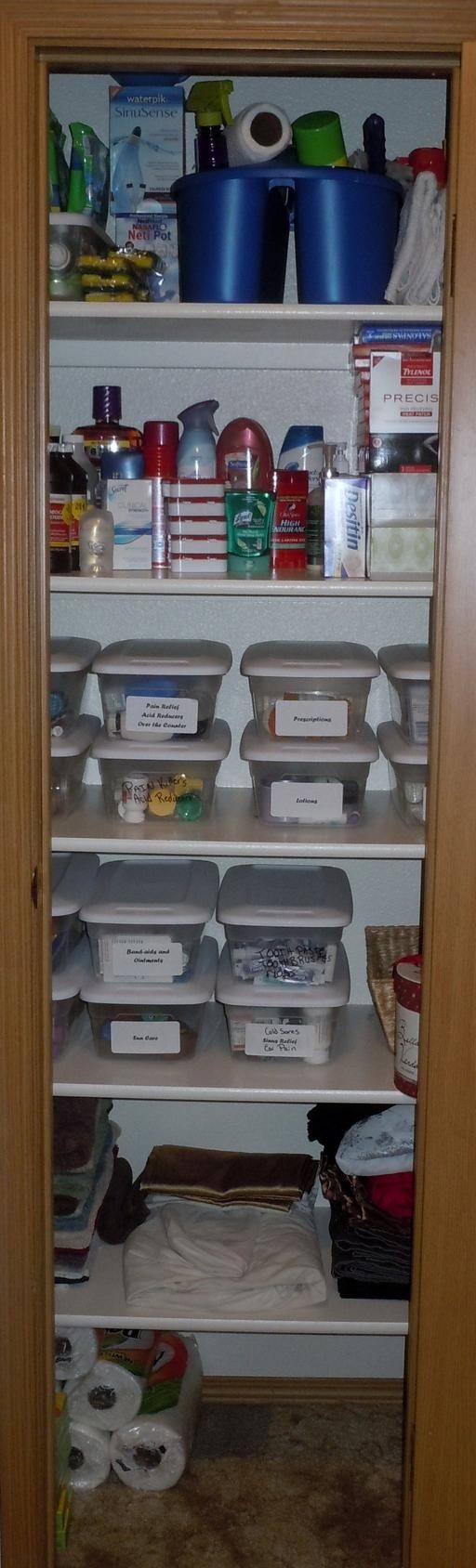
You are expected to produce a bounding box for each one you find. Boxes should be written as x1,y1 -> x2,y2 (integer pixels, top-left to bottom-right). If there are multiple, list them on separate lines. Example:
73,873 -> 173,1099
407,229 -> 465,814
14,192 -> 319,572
106,711 -> 174,741
52,722 -> 64,737
79,508 -> 115,576
122,775 -> 148,823
279,772 -> 358,804
57,442 -> 88,572
47,440 -> 88,573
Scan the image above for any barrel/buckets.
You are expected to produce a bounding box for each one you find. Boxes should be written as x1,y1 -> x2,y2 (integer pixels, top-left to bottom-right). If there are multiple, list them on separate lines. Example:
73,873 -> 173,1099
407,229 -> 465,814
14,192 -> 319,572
169,166 -> 289,307
291,170 -> 405,307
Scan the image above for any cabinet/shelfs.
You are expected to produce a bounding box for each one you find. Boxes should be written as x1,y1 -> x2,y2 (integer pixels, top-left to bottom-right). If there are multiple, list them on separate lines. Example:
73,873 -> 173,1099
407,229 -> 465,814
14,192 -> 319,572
40,53 -> 451,1562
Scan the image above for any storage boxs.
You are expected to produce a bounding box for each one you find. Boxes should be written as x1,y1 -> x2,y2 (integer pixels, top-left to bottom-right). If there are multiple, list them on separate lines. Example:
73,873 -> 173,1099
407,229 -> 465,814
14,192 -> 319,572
79,856 -> 221,985
49,714 -> 101,818
47,212 -> 117,303
376,720 -> 424,826
239,642 -> 381,747
49,634 -> 102,735
377,642 -> 432,746
78,933 -> 217,1060
239,718 -> 379,828
51,937 -> 92,1064
90,639 -> 232,745
213,942 -> 350,1067
89,717 -> 232,823
216,863 -> 353,987
51,850 -> 98,977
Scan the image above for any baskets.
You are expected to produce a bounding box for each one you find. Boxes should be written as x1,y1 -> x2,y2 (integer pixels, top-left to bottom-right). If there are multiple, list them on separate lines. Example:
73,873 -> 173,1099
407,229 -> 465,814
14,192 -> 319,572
365,926 -> 423,1055
